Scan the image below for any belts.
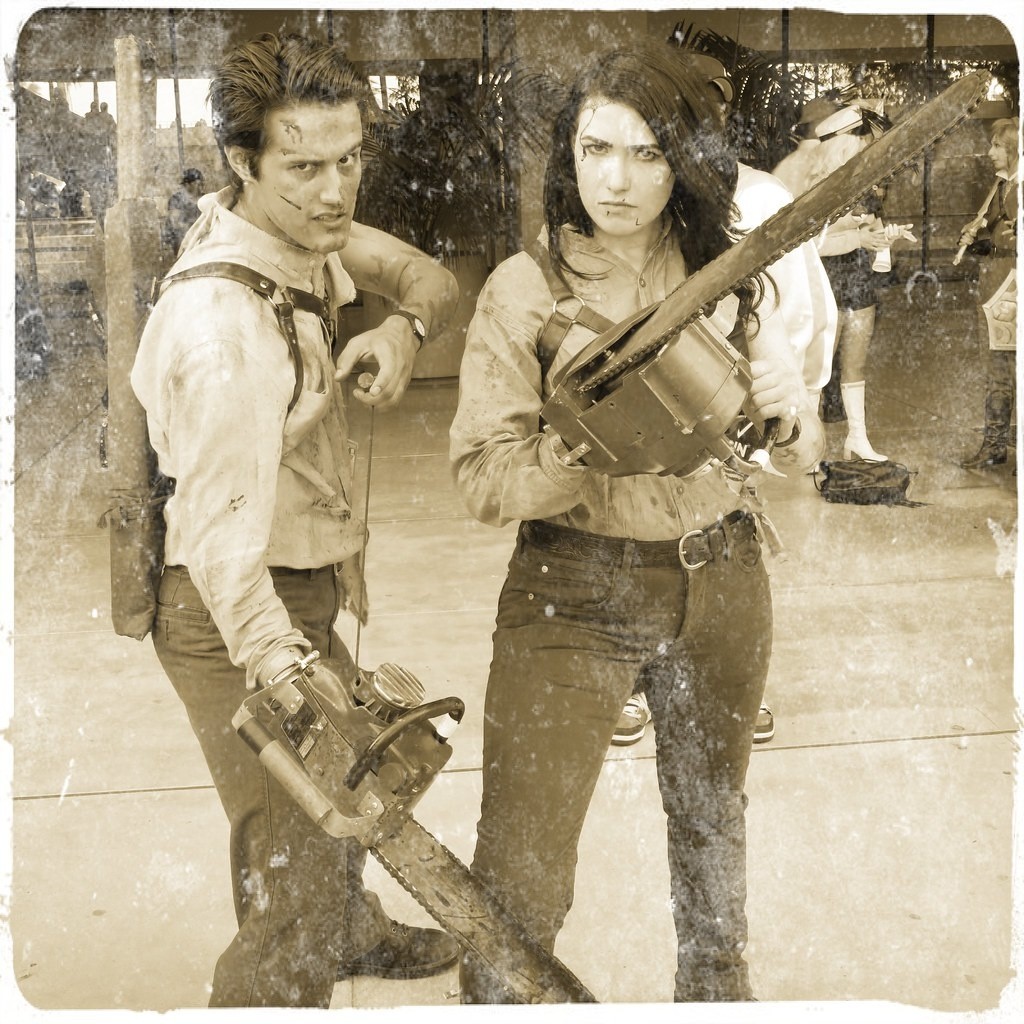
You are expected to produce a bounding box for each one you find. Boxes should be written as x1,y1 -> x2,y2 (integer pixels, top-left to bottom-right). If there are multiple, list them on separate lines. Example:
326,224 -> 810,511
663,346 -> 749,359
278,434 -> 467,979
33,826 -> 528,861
173,563 -> 324,576
821,257 -> 867,270
989,247 -> 1016,258
523,508 -> 758,568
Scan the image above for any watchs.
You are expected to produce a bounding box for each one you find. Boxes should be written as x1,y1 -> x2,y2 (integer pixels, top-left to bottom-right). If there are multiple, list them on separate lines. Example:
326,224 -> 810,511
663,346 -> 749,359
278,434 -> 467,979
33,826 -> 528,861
390,309 -> 428,352
774,416 -> 803,448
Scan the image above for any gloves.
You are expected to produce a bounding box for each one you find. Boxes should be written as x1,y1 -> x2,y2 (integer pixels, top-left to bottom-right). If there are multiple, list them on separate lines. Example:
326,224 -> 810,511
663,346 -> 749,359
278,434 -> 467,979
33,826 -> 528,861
872,227 -> 917,272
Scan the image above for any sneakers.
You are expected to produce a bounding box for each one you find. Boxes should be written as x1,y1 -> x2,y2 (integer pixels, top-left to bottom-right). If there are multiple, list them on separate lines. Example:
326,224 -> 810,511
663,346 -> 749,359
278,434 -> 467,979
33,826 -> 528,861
611,692 -> 652,745
754,702 -> 775,743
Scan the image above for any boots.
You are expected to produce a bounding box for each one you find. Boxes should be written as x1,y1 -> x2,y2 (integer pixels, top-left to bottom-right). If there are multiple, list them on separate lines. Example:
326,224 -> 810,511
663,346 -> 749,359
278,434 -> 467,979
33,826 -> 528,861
823,369 -> 846,422
841,381 -> 888,462
961,409 -> 1012,469
808,391 -> 820,475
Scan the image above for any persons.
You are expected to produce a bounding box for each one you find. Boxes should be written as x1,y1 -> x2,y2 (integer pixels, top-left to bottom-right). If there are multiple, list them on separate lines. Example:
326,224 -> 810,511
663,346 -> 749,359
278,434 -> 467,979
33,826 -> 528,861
163,168 -> 203,259
958,119 -> 1016,476
133,32 -> 463,1007
772,84 -> 902,472
448,34 -> 838,1003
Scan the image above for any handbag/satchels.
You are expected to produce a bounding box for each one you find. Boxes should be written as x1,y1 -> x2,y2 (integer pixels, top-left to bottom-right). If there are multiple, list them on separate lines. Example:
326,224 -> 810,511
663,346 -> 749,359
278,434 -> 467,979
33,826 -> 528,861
820,459 -> 914,504
108,498 -> 162,638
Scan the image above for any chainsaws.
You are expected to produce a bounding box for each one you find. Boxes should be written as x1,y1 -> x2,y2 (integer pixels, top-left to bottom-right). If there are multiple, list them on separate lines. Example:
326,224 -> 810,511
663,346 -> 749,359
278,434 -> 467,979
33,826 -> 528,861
539,68 -> 994,482
231,649 -> 598,1004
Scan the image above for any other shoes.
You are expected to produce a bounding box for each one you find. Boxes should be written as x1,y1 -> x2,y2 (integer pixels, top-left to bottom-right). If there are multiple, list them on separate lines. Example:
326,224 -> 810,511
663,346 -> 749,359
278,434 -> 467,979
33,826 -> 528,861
334,920 -> 459,983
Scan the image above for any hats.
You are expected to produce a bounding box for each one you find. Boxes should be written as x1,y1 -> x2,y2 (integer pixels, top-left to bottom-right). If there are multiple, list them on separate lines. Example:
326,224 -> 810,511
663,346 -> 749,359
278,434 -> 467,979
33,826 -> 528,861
799,97 -> 838,126
691,52 -> 735,102
180,169 -> 203,184
815,104 -> 871,142
